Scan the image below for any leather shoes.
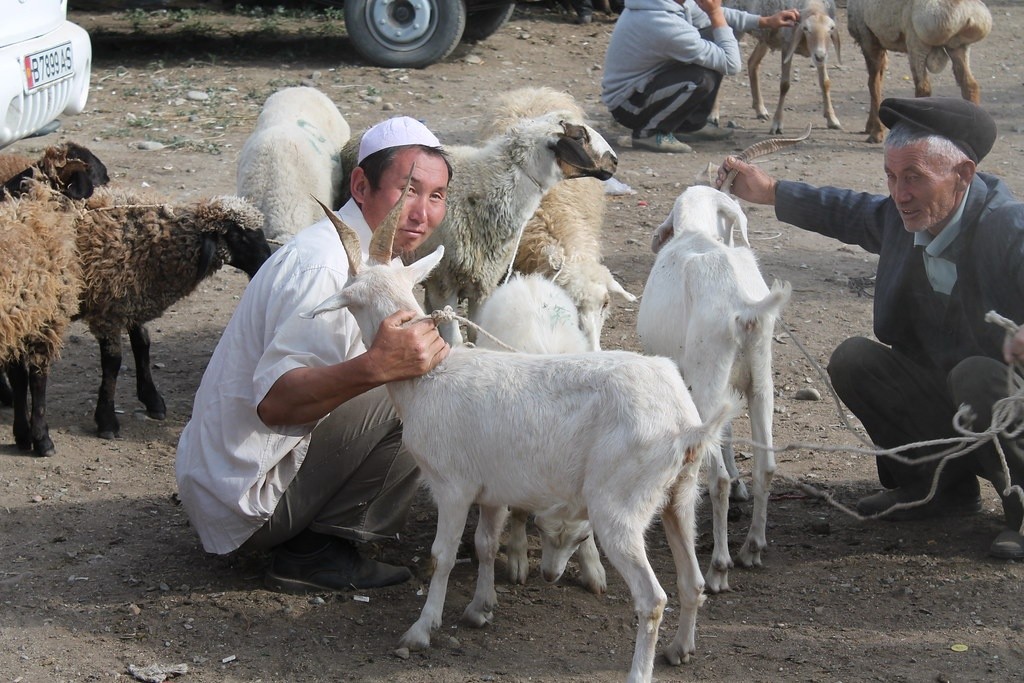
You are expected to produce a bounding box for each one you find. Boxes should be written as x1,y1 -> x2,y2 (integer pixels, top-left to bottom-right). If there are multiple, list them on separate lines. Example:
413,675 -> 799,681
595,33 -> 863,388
265,540 -> 410,594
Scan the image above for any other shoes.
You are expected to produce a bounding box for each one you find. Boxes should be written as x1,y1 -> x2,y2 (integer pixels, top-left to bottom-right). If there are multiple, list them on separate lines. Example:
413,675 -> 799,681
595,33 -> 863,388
675,122 -> 733,138
632,132 -> 690,152
858,476 -> 980,518
990,527 -> 1024,558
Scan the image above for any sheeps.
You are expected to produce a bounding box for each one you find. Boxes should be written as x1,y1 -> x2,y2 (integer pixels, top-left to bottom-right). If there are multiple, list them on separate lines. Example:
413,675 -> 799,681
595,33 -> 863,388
299,160 -> 728,683
632,123 -> 814,592
721,0 -> 845,137
834,1 -> 993,144
235,87 -> 639,351
0,141 -> 273,458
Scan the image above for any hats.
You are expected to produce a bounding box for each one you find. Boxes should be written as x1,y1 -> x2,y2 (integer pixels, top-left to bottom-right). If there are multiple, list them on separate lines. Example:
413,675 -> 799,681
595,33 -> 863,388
880,98 -> 996,166
359,117 -> 442,164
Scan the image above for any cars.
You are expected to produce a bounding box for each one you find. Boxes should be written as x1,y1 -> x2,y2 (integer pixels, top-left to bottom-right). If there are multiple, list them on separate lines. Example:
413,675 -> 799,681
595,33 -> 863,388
0,0 -> 94,148
344,0 -> 517,69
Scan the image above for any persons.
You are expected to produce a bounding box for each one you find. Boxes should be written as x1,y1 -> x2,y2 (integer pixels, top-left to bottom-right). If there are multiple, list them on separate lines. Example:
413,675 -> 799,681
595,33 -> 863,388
716,96 -> 1024,558
600,0 -> 800,153
174,115 -> 450,595
